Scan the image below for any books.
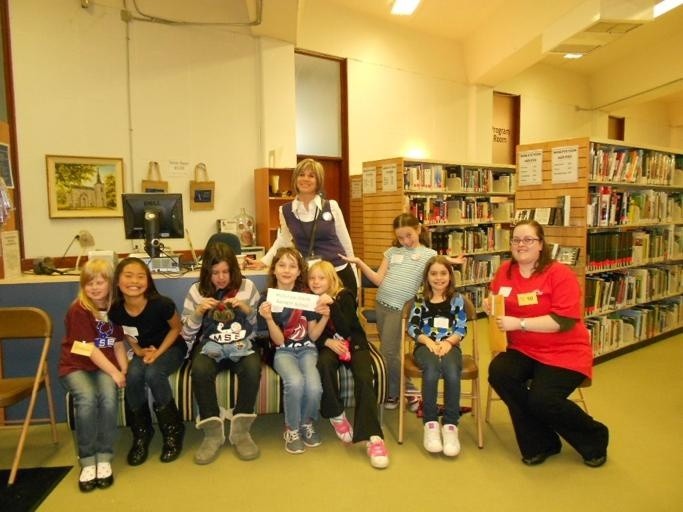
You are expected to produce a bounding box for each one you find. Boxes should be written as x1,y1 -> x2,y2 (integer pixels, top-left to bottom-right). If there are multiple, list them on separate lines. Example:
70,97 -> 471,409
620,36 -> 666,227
584,142 -> 683,359
404,165 -> 580,309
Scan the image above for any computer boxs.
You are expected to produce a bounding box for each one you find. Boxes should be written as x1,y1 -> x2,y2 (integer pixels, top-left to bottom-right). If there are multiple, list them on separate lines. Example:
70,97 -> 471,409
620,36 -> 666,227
129,253 -> 182,273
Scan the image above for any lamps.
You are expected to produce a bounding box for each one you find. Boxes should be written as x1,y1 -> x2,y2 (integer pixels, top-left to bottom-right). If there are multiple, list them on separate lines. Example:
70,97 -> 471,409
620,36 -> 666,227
50,231 -> 95,275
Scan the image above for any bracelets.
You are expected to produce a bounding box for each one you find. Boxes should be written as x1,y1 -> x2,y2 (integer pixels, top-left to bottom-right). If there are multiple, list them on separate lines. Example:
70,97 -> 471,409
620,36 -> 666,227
444,339 -> 453,346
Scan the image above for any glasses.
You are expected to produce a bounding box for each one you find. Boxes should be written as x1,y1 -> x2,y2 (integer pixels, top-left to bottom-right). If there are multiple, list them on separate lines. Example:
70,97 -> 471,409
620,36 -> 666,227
509,236 -> 540,245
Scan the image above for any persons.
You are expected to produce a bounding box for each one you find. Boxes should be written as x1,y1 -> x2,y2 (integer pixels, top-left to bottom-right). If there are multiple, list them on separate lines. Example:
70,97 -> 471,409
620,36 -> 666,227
338,214 -> 437,412
305,261 -> 390,468
246,158 -> 360,306
181,241 -> 262,465
258,247 -> 331,455
57,256 -> 128,492
107,258 -> 188,466
489,220 -> 609,466
408,256 -> 468,457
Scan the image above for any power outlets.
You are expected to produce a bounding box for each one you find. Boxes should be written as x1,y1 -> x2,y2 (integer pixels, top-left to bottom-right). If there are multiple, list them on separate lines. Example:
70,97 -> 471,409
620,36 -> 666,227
133,240 -> 144,251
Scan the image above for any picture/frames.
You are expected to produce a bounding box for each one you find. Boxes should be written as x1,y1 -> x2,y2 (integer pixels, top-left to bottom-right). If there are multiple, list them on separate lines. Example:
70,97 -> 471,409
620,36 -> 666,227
45,154 -> 125,218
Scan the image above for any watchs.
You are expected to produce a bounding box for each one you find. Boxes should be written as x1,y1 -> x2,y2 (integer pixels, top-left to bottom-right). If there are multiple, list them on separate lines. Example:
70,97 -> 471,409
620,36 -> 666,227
521,319 -> 525,328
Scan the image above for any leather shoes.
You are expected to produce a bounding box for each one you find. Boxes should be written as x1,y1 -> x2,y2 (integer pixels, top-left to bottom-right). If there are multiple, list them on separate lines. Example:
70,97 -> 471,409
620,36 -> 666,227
586,428 -> 609,466
97,461 -> 113,489
79,465 -> 96,492
522,435 -> 561,465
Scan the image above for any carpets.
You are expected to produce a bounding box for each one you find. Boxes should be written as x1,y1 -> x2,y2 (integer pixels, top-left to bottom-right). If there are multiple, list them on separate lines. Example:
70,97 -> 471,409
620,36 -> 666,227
0,465 -> 74,512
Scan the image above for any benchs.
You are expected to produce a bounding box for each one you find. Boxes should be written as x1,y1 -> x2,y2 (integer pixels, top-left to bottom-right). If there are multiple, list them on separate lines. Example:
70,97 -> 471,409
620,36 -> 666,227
64,331 -> 387,464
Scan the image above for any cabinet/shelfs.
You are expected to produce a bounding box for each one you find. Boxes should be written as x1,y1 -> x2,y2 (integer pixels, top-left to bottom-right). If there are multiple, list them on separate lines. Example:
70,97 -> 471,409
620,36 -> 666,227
0,268 -> 276,425
254,167 -> 294,249
362,157 -> 515,314
516,136 -> 683,367
241,246 -> 265,260
349,174 -> 362,263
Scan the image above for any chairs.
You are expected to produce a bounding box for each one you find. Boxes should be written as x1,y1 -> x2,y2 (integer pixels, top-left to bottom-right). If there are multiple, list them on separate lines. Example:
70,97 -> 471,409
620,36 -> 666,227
485,294 -> 591,422
397,297 -> 484,450
0,306 -> 58,489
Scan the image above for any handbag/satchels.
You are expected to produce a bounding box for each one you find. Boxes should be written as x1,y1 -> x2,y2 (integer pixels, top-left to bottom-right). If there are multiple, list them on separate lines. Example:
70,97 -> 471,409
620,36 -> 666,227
141,162 -> 167,194
190,163 -> 215,210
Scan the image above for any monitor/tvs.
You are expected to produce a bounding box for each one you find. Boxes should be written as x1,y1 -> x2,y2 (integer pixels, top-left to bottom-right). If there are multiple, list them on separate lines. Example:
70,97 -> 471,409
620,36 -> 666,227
121,193 -> 184,258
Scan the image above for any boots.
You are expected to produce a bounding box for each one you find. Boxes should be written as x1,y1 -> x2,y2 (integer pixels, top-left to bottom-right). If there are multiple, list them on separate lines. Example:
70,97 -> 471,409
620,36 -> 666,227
194,406 -> 225,464
225,408 -> 260,461
127,399 -> 154,466
152,399 -> 184,462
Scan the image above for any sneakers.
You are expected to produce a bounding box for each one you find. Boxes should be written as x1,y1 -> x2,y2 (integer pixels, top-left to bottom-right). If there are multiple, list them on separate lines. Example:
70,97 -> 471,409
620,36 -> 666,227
385,397 -> 399,410
329,411 -> 354,443
300,424 -> 322,447
442,424 -> 460,456
423,421 -> 443,453
406,389 -> 421,412
367,435 -> 389,469
284,431 -> 306,454
